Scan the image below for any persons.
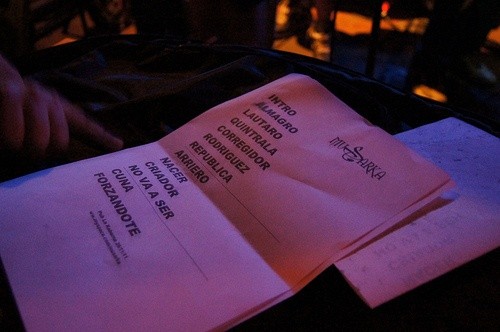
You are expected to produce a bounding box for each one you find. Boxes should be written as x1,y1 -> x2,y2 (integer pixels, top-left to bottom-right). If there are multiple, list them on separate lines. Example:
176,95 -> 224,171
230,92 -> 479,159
0,58 -> 128,155
